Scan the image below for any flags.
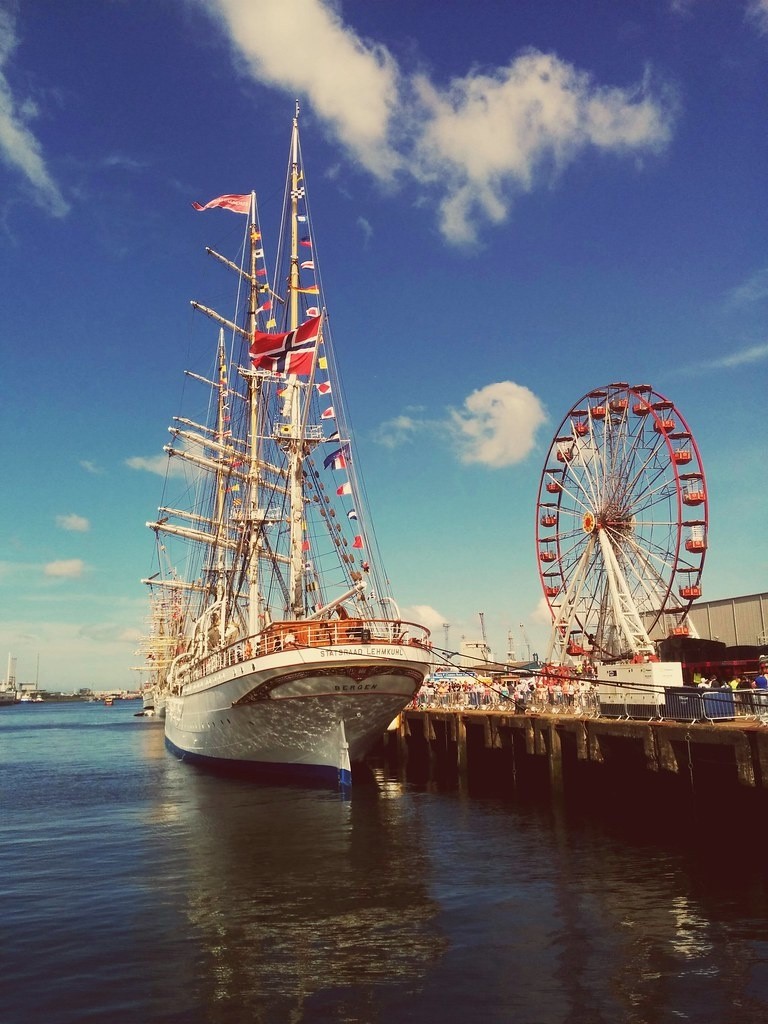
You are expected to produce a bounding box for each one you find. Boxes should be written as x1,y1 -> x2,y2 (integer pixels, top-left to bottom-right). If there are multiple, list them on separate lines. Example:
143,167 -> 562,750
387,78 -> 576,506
248,169 -> 377,614
218,353 -> 245,491
191,193 -> 251,214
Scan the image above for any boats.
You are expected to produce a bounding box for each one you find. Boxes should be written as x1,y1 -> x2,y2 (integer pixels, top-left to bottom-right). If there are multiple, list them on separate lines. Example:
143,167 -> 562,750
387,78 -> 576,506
105,697 -> 114,706
22,689 -> 34,703
32,695 -> 44,703
0,653 -> 21,705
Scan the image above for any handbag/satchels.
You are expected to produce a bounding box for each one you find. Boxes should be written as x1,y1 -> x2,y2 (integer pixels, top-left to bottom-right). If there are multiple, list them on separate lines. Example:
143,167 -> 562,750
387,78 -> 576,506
520,690 -> 524,695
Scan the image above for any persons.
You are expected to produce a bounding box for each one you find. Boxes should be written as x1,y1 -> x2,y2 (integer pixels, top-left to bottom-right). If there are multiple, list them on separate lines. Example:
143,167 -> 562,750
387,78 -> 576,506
417,678 -> 586,714
697,670 -> 768,715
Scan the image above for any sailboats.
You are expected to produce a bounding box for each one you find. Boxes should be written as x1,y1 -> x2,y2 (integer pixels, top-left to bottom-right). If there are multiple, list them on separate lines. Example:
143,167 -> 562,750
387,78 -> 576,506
143,93 -> 436,778
132,565 -> 196,718
